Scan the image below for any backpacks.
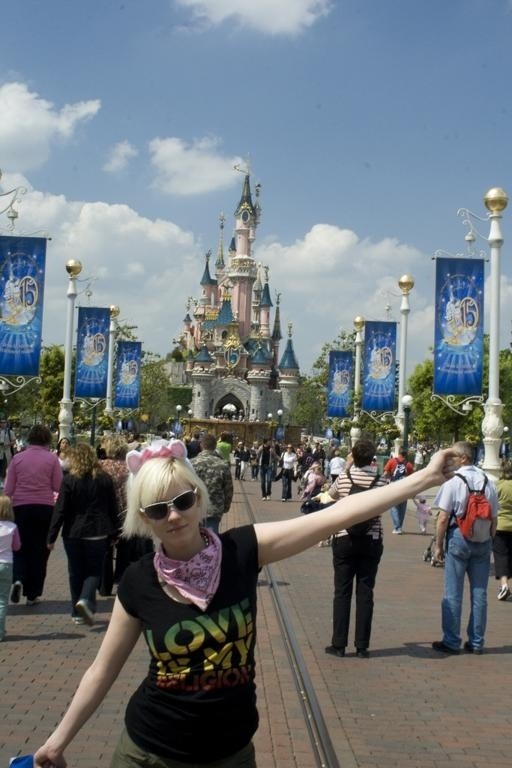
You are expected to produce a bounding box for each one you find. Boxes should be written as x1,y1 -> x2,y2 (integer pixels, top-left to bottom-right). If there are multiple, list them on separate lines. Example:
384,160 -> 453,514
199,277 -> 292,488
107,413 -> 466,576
392,457 -> 409,481
345,469 -> 381,537
451,474 -> 492,543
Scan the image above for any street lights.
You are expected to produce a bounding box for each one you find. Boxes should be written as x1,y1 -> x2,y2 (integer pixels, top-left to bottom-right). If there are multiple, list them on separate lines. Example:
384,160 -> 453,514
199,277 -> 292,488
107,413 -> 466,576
398,393 -> 414,463
88,393 -> 100,445
174,402 -> 286,434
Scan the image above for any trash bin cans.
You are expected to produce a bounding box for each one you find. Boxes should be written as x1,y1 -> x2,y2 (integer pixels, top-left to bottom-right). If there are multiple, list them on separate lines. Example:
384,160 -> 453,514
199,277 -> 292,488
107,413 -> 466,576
377,456 -> 390,475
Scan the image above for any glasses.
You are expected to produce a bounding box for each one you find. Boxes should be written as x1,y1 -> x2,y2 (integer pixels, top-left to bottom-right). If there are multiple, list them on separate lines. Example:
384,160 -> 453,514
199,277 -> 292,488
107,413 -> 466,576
139,486 -> 198,520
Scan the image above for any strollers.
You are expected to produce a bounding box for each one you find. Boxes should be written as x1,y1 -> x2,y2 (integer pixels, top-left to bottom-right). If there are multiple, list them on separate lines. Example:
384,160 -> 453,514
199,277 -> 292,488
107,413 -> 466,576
424,513 -> 449,569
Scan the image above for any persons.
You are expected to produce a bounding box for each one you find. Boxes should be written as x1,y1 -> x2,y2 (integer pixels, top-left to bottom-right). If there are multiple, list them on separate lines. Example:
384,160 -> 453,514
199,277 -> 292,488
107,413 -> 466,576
45,442 -> 120,626
433,439 -> 499,654
492,457 -> 512,601
6,424 -> 64,605
1,420 -> 433,547
96,444 -> 128,596
316,438 -> 391,658
23,435 -> 461,768
1,496 -> 22,642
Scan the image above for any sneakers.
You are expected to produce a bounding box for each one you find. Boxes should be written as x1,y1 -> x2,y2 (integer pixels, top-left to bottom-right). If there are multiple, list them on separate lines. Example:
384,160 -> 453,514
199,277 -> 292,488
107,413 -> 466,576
433,641 -> 457,655
392,526 -> 402,534
498,585 -> 512,600
27,598 -> 40,605
464,640 -> 482,653
325,645 -> 345,658
356,648 -> 368,658
10,581 -> 23,602
72,599 -> 96,625
